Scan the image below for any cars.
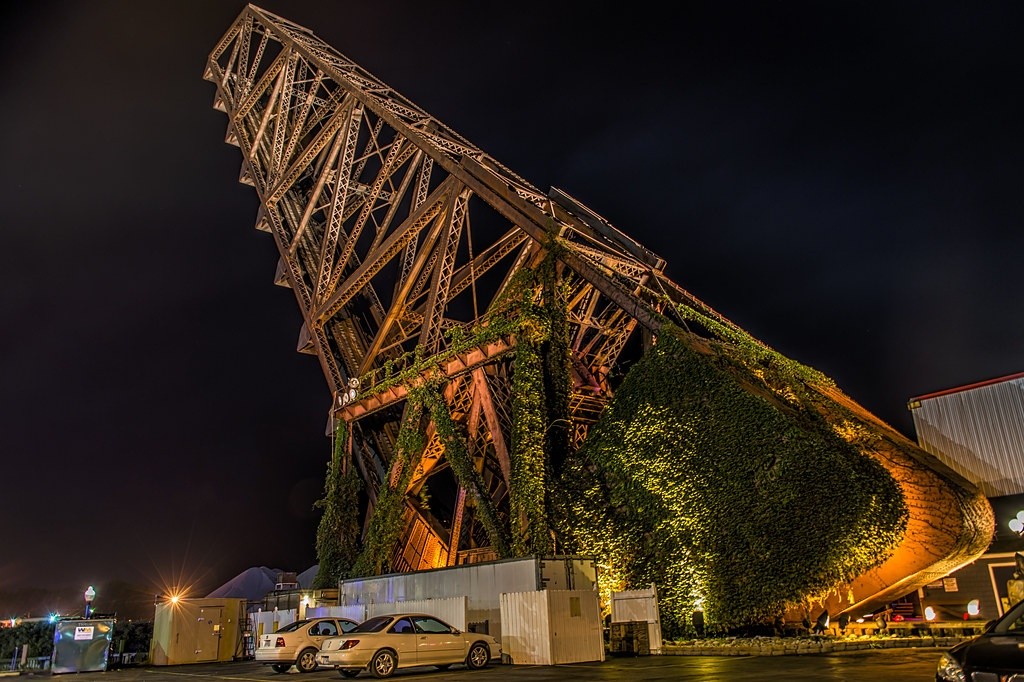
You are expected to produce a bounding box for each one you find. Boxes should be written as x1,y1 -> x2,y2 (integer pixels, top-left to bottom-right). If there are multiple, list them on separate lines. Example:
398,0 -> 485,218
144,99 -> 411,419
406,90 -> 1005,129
255,616 -> 362,673
937,599 -> 1024,682
315,611 -> 504,678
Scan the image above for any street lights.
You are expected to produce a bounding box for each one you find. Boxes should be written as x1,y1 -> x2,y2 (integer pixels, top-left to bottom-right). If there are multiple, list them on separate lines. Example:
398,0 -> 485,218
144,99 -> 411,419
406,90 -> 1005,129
85,586 -> 96,620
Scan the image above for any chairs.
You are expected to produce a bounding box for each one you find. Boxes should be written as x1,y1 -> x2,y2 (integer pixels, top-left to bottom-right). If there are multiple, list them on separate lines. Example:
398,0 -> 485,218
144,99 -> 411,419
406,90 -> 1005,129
322,629 -> 330,635
402,626 -> 412,633
310,628 -> 320,635
